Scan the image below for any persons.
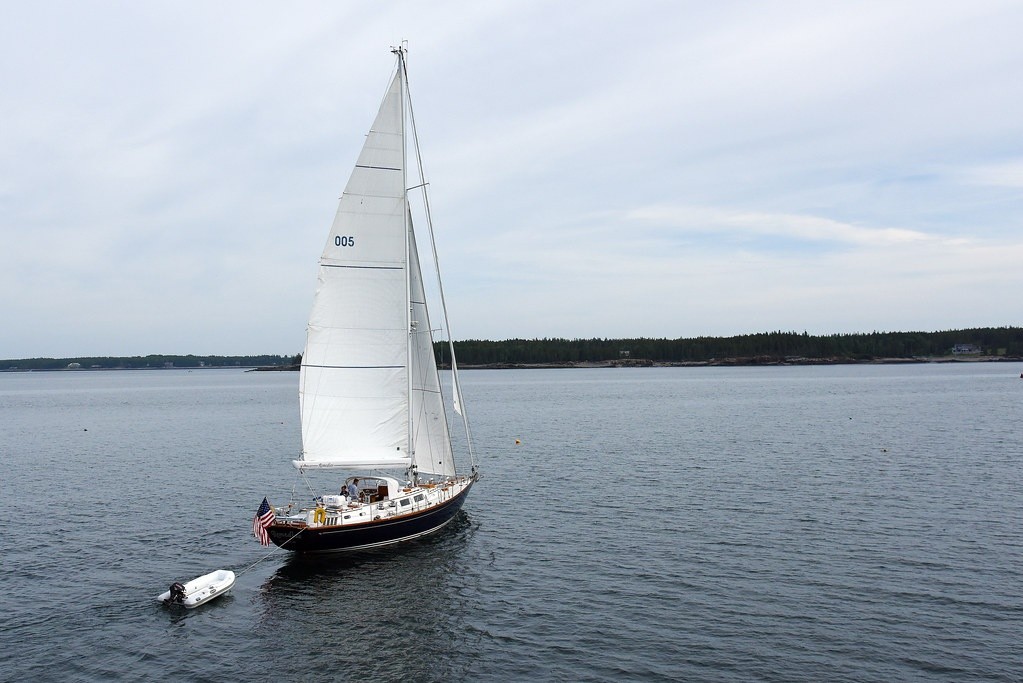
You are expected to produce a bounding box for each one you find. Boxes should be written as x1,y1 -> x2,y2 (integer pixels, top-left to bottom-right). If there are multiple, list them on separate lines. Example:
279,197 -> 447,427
340,484 -> 349,498
349,479 -> 361,500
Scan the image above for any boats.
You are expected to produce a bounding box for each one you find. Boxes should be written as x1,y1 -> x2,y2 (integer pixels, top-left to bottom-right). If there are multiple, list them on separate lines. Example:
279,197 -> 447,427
157,569 -> 236,610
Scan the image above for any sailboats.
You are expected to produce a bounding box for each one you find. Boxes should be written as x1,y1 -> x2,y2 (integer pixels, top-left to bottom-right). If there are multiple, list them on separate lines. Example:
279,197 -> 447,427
257,40 -> 481,554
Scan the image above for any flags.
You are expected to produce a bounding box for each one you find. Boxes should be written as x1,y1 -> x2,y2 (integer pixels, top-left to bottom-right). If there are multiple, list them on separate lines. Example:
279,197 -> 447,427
254,497 -> 276,547
